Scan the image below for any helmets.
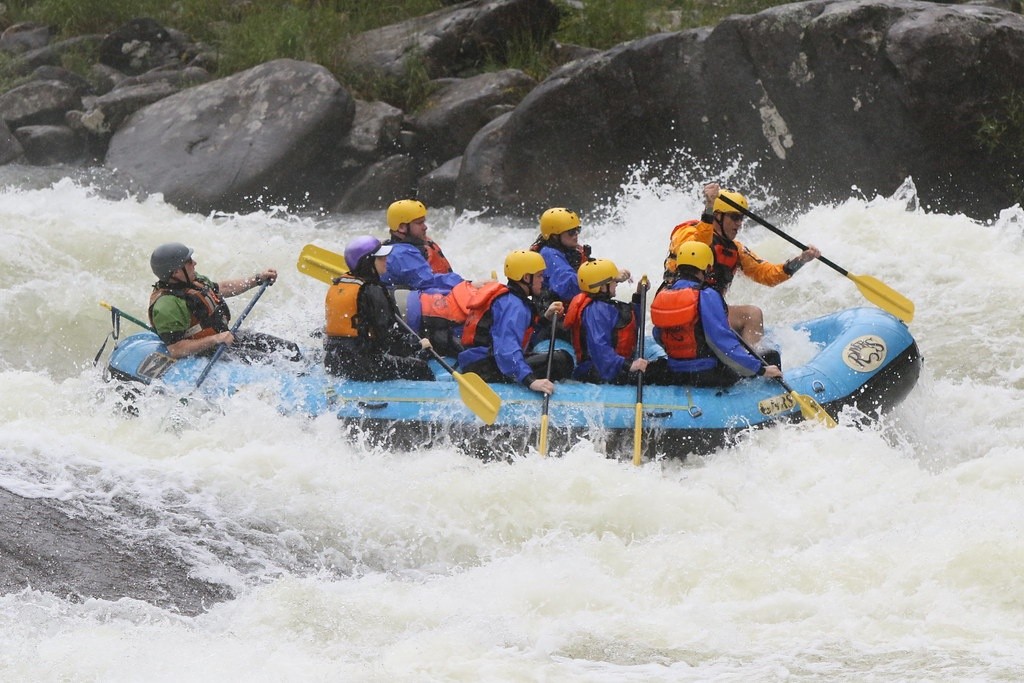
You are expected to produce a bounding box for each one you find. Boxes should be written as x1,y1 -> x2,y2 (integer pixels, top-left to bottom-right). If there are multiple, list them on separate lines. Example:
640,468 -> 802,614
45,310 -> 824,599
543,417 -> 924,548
713,188 -> 749,214
677,241 -> 714,270
539,208 -> 581,241
345,235 -> 381,274
577,260 -> 619,293
505,251 -> 547,281
387,199 -> 427,232
150,242 -> 194,282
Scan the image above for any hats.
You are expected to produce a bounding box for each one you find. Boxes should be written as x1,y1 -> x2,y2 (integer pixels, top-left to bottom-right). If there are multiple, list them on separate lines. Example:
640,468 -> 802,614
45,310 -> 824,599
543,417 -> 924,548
371,246 -> 393,258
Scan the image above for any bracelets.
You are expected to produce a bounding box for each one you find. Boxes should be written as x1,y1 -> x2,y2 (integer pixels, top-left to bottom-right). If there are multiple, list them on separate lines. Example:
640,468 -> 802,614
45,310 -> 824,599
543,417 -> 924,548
256,274 -> 262,285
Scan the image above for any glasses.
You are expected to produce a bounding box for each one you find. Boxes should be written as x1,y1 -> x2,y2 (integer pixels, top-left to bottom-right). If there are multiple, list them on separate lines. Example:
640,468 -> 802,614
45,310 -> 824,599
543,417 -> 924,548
565,227 -> 582,237
723,213 -> 744,220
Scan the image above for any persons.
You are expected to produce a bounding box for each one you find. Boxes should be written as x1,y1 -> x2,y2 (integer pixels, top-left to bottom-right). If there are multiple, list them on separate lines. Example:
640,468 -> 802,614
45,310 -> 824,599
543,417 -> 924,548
148,243 -> 299,363
460,181 -> 820,395
324,236 -> 436,382
376,199 -> 497,290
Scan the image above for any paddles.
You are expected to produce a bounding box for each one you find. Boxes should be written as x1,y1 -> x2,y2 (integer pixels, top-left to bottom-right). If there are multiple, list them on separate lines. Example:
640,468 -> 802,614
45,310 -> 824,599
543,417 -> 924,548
178,270 -> 272,408
295,243 -> 357,285
730,324 -> 837,427
632,273 -> 649,467
98,299 -> 161,337
713,191 -> 915,323
386,310 -> 504,426
537,302 -> 560,463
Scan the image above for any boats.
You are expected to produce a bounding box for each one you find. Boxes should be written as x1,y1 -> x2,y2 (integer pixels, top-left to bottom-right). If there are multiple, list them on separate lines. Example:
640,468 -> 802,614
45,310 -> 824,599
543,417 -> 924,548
91,303 -> 923,464
